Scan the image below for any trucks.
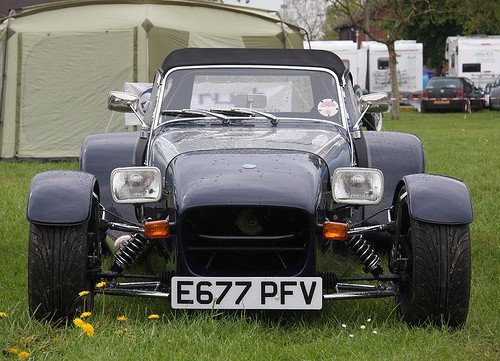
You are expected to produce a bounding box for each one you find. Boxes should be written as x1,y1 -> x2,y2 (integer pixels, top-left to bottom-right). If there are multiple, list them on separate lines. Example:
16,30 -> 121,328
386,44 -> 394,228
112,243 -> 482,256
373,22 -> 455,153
302,40 -> 423,113
445,34 -> 500,108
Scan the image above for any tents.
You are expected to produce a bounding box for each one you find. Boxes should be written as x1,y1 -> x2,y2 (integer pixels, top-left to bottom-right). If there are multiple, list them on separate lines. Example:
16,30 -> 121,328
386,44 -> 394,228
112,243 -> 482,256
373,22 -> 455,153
0,1 -> 310,164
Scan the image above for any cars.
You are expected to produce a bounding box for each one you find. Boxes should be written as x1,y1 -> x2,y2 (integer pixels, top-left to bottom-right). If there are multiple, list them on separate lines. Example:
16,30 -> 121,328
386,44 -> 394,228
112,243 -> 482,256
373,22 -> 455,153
421,75 -> 488,114
487,75 -> 499,110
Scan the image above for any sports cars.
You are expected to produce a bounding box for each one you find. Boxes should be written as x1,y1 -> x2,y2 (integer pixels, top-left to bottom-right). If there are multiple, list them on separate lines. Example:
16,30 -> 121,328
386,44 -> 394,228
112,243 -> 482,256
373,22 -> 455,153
28,47 -> 473,329
